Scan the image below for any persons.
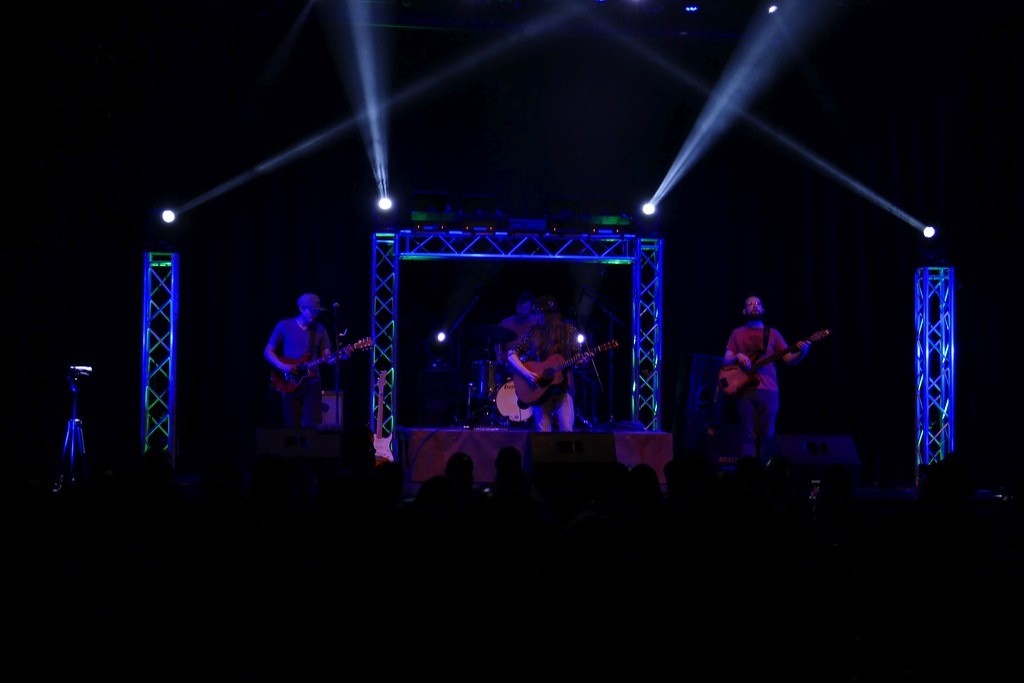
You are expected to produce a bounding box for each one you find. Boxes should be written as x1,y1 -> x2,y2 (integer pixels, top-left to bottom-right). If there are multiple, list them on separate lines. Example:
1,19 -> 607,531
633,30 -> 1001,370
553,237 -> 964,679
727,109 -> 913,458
508,298 -> 591,433
10,449 -> 1022,683
724,293 -> 812,466
265,293 -> 339,428
496,296 -> 540,354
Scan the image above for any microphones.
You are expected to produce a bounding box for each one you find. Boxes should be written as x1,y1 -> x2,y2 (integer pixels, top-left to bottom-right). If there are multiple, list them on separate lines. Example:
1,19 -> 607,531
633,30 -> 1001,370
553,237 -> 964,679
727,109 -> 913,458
330,302 -> 341,334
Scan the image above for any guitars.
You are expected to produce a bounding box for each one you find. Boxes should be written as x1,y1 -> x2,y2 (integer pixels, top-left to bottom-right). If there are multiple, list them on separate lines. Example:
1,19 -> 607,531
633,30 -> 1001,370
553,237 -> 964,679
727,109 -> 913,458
515,339 -> 620,402
267,337 -> 373,391
717,326 -> 834,394
367,370 -> 394,467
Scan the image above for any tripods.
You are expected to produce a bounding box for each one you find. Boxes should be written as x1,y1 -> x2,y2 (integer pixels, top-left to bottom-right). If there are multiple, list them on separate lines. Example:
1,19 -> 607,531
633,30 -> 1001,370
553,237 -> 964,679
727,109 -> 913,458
57,417 -> 90,485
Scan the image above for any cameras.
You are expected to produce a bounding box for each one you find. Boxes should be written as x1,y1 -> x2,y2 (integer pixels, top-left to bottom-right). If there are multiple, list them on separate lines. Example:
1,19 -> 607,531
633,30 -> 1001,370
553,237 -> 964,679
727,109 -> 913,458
69,365 -> 94,379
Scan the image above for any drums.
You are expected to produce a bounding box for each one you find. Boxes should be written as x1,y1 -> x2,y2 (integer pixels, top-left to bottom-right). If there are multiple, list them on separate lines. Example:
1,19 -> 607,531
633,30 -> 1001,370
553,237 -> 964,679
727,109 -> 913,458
470,358 -> 537,422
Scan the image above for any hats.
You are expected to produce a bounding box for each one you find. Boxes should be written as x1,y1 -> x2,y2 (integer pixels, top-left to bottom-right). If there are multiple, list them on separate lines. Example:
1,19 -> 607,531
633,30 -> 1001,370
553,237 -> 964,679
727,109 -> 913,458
298,293 -> 327,314
530,297 -> 563,316
